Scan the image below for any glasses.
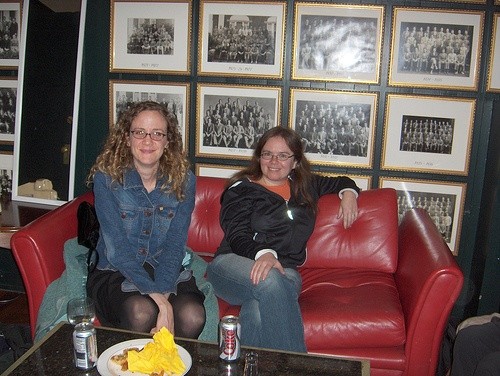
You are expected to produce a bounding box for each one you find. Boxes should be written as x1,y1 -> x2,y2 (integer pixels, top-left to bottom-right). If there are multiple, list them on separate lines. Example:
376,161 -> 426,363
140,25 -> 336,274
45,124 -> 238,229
130,129 -> 166,141
260,152 -> 294,161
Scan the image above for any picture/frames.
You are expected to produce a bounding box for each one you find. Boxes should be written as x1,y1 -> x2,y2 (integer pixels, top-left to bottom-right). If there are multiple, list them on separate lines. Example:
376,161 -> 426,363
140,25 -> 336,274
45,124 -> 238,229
195,163 -> 252,179
287,85 -> 379,169
110,1 -> 191,76
106,80 -> 191,159
379,176 -> 468,256
378,93 -> 477,177
387,5 -> 485,92
290,1 -> 387,84
196,83 -> 284,159
198,0 -> 288,79
486,12 -> 500,94
311,172 -> 372,193
0,0 -> 24,193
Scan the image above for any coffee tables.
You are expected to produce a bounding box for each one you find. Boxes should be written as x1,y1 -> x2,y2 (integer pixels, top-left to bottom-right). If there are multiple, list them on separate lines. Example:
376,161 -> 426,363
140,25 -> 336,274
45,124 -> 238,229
0,321 -> 372,376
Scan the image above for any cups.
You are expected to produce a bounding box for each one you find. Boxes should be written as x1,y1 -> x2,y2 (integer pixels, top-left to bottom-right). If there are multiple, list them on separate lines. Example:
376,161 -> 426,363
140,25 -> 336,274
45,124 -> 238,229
242,352 -> 259,376
66,295 -> 96,326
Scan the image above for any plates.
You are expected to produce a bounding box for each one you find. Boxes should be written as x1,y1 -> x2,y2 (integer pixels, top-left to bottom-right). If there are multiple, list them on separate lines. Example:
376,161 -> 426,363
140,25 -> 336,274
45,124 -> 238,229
96,339 -> 193,376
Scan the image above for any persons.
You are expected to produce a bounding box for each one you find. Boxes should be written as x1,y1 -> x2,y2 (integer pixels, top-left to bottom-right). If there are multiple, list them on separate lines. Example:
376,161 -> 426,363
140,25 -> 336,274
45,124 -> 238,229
401,27 -> 470,75
296,105 -> 369,156
300,19 -> 374,68
207,126 -> 360,354
401,119 -> 453,153
86,102 -> 207,340
208,25 -> 274,64
0,89 -> 17,134
128,23 -> 171,54
397,196 -> 452,239
115,96 -> 184,127
0,169 -> 12,201
0,16 -> 18,55
203,98 -> 274,148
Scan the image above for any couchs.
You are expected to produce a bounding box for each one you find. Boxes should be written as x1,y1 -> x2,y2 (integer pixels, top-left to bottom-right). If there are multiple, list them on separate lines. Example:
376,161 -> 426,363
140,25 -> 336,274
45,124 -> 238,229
10,174 -> 464,376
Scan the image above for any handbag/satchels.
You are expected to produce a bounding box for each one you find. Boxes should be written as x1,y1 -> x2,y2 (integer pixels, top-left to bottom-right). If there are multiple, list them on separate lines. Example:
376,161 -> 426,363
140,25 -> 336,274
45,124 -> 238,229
77,200 -> 100,274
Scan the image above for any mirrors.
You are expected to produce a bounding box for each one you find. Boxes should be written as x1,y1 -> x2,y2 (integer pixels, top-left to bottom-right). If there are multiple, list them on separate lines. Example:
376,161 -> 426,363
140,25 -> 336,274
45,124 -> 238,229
12,0 -> 87,205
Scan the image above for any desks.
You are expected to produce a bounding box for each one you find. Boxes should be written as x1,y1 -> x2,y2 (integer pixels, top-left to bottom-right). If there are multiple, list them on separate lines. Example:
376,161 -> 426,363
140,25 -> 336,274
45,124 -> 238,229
0,195 -> 51,232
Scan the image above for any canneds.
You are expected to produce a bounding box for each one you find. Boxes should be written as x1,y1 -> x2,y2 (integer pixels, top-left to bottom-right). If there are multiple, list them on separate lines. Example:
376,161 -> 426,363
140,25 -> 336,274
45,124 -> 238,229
218,315 -> 241,362
217,363 -> 240,376
72,322 -> 98,370
